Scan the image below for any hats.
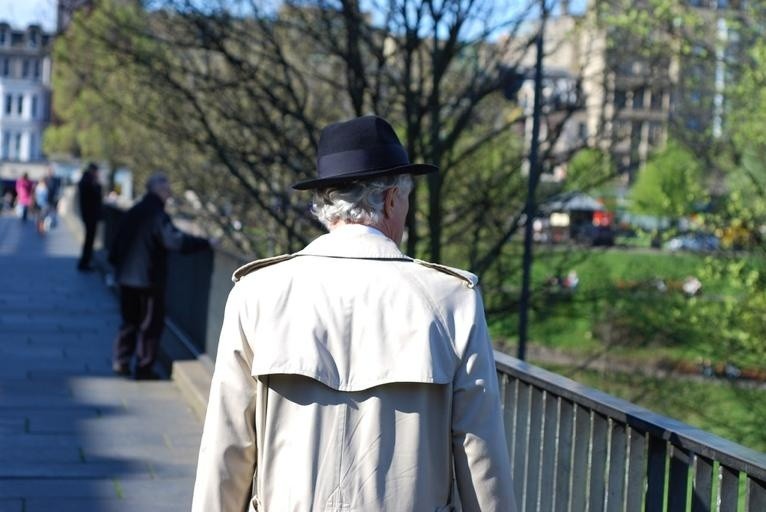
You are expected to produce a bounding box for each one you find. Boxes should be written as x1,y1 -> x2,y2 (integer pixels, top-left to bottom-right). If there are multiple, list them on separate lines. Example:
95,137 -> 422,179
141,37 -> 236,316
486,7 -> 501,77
293,115 -> 439,190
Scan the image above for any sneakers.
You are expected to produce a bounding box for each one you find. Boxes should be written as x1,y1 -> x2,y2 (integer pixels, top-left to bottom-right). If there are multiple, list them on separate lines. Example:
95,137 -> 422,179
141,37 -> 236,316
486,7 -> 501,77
113,363 -> 162,380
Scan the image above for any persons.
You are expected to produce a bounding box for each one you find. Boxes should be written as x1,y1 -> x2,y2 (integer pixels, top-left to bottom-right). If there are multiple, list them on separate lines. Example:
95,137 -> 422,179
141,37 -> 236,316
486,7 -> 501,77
42,165 -> 62,230
73,161 -> 103,273
33,175 -> 50,238
104,172 -> 221,380
14,170 -> 32,225
186,112 -> 520,512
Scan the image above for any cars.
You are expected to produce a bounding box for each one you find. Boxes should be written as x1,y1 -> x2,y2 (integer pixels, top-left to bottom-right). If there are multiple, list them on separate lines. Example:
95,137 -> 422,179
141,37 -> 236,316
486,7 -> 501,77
574,224 -> 616,246
659,228 -> 719,252
539,271 -> 582,301
